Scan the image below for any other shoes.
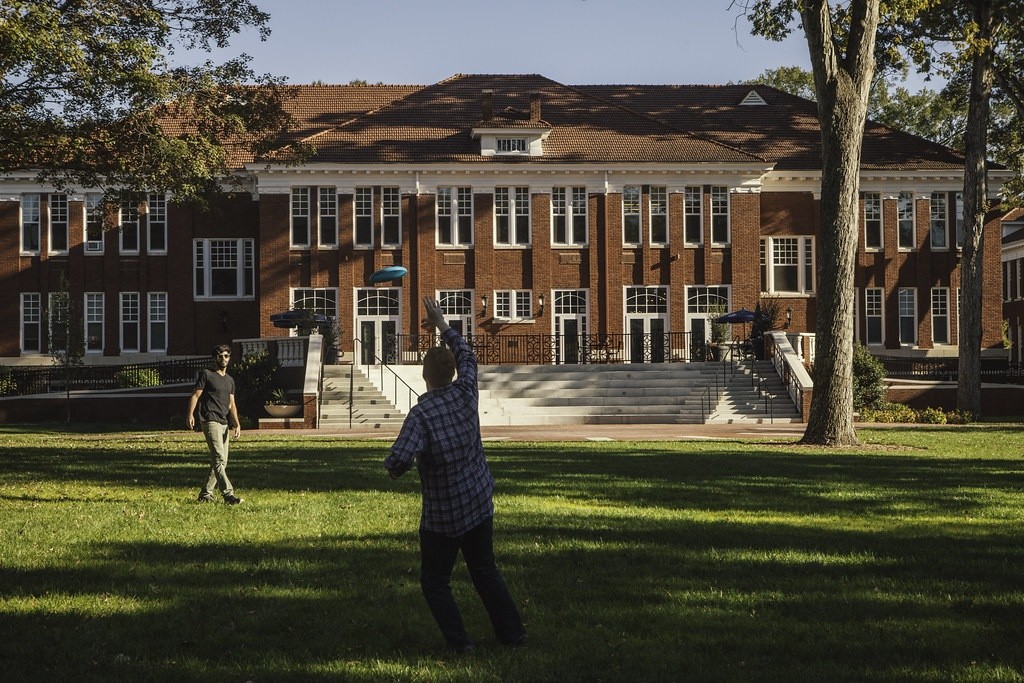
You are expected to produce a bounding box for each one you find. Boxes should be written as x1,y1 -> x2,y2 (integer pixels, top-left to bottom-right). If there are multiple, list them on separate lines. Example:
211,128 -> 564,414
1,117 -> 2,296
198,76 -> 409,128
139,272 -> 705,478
511,633 -> 530,644
442,640 -> 476,654
225,496 -> 239,504
199,494 -> 214,502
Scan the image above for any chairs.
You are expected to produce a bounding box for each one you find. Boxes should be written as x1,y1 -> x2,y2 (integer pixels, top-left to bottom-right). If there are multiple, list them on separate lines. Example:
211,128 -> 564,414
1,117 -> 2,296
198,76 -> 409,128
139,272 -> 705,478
606,340 -> 621,364
577,341 -> 592,364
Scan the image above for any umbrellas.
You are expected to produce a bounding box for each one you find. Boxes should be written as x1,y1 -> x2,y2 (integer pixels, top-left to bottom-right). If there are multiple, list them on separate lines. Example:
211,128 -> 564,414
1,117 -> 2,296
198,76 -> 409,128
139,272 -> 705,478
711,307 -> 756,344
270,309 -> 331,336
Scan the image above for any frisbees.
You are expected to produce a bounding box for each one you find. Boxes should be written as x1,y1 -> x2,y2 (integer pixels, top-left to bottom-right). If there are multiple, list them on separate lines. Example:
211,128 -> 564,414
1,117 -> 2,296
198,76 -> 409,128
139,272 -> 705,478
370,264 -> 407,282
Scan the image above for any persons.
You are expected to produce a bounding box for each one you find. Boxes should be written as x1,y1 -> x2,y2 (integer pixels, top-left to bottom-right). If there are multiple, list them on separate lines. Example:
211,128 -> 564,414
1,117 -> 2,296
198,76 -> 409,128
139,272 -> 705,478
185,345 -> 241,504
384,296 -> 531,651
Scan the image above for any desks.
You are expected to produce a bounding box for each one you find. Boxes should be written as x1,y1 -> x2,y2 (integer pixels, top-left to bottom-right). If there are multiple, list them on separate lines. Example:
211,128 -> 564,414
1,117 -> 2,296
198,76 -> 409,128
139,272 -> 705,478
589,344 -> 608,364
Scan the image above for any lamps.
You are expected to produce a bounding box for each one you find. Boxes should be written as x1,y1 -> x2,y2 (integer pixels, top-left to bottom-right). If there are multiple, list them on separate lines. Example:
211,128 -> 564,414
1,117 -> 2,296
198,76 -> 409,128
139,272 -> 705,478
786,306 -> 793,325
481,294 -> 487,314
538,293 -> 546,314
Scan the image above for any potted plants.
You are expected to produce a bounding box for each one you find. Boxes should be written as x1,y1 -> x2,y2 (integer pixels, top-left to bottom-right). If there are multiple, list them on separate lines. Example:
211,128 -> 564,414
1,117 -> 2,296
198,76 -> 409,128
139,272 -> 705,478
703,296 -> 730,361
317,311 -> 337,365
263,388 -> 303,417
